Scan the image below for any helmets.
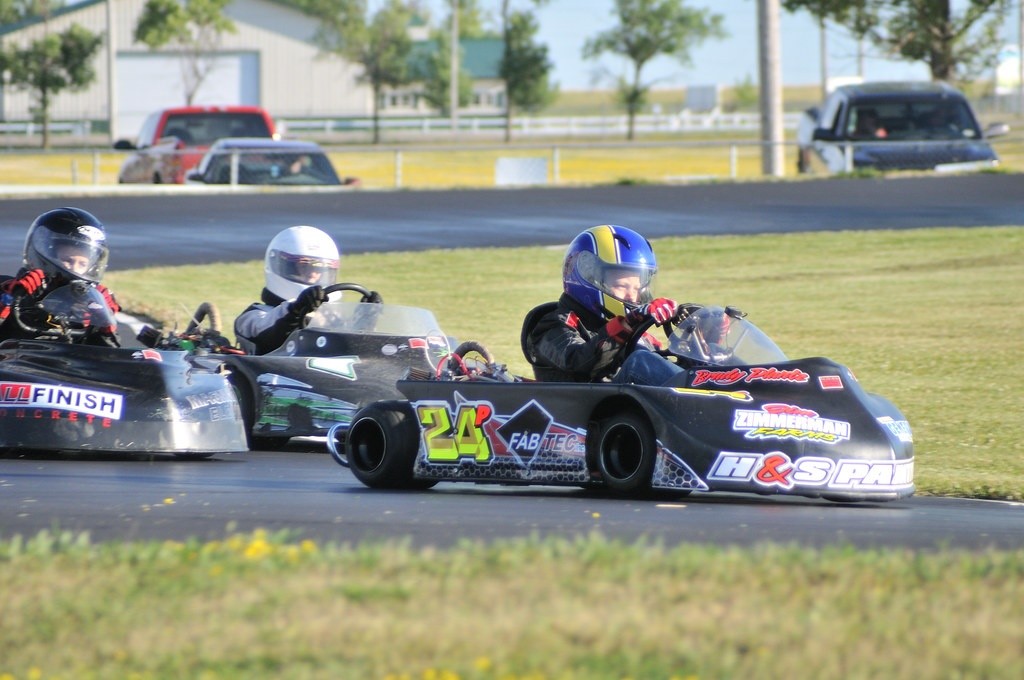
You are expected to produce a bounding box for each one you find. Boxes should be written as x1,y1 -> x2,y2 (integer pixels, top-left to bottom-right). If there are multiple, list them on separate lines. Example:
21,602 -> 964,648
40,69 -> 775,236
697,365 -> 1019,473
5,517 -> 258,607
562,225 -> 659,323
22,207 -> 109,288
261,226 -> 340,303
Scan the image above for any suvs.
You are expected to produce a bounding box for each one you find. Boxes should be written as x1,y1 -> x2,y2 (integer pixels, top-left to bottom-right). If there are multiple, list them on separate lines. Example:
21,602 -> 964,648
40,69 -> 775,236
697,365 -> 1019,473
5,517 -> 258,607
795,79 -> 1011,177
113,103 -> 283,184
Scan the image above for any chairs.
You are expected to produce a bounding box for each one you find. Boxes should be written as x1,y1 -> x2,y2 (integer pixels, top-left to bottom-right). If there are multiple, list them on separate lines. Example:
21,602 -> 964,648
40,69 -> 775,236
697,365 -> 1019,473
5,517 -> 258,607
521,302 -> 561,364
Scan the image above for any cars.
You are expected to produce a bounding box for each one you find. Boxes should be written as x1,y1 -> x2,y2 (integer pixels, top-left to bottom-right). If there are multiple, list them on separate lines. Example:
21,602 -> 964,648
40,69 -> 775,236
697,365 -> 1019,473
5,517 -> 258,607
184,137 -> 363,185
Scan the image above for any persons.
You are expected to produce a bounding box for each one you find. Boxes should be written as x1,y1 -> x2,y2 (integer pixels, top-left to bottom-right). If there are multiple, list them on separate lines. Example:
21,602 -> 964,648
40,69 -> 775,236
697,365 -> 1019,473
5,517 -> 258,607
233,224 -> 384,357
852,108 -> 889,140
284,155 -> 315,173
0,207 -> 125,345
522,225 -> 731,388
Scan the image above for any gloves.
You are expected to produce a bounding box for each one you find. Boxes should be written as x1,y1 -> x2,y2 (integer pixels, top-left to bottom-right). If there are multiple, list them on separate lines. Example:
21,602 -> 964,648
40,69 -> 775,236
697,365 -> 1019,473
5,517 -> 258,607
713,309 -> 730,336
294,285 -> 329,314
7,267 -> 47,302
357,291 -> 384,315
94,284 -> 119,316
628,297 -> 678,328
